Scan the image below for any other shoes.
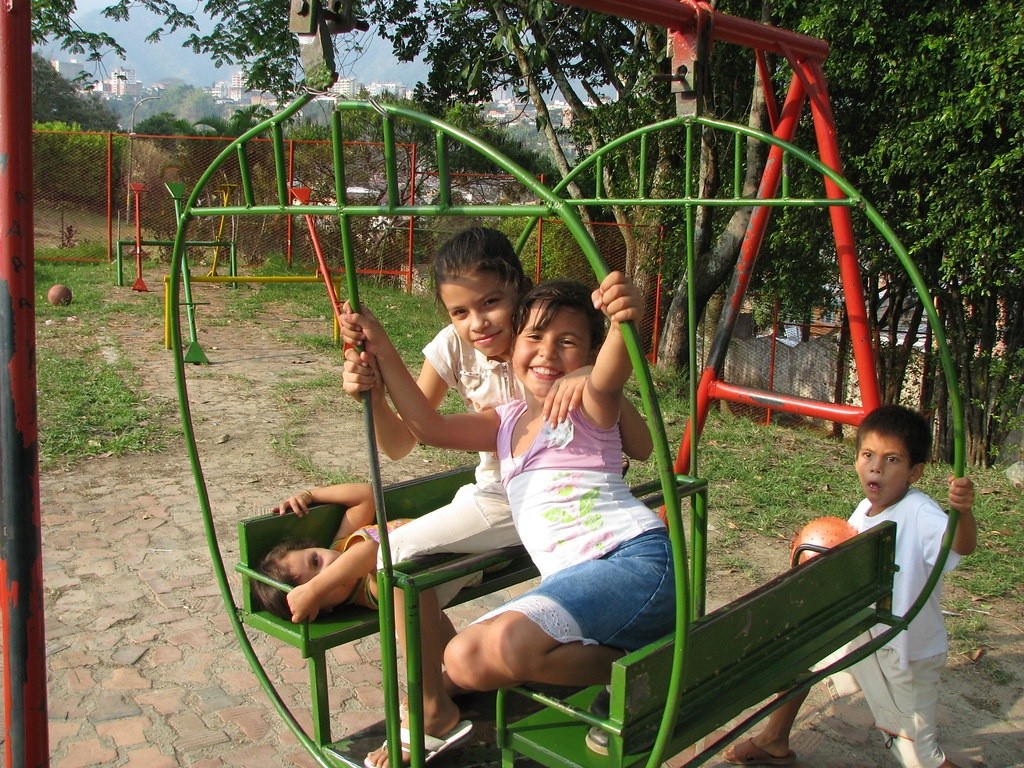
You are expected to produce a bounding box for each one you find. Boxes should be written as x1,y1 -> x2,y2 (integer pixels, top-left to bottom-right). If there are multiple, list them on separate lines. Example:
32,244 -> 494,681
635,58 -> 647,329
585,726 -> 656,756
586,684 -> 610,718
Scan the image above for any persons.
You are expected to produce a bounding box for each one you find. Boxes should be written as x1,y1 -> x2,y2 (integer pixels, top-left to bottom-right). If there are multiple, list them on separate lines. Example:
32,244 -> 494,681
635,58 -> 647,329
721,403 -> 978,768
339,223 -> 656,768
338,269 -> 677,756
249,451 -> 630,624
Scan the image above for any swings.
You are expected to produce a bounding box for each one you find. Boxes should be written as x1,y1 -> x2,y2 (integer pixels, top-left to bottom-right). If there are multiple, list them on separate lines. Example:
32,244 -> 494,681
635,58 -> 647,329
161,1 -> 973,768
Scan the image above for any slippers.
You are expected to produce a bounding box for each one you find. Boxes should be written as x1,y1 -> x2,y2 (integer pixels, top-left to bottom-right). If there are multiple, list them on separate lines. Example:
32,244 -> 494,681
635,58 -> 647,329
363,719 -> 474,768
399,664 -> 475,720
721,737 -> 797,765
